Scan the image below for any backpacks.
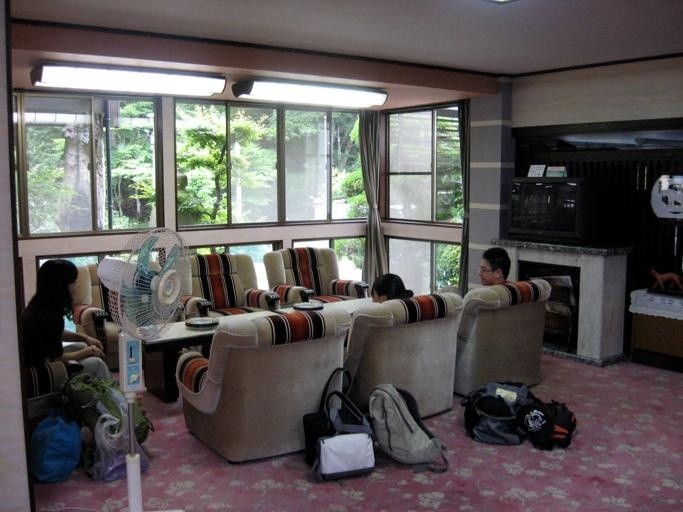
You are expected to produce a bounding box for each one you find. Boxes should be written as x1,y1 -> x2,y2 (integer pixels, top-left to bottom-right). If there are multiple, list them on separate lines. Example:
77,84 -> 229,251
299,368 -> 449,484
28,370 -> 154,484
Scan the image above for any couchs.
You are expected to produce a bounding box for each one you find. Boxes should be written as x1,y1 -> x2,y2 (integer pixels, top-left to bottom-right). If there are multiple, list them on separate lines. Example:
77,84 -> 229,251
69,259 -> 212,371
454,277 -> 550,398
175,310 -> 350,464
341,292 -> 463,420
262,248 -> 370,310
176,252 -> 280,319
24,361 -> 70,440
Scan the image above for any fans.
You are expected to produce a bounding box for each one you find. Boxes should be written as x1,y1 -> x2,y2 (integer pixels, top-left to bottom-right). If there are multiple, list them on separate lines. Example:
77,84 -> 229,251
98,226 -> 189,512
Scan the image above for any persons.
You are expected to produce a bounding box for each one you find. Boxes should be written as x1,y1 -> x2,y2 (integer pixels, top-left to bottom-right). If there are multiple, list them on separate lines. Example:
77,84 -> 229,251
479,247 -> 511,287
371,273 -> 417,302
23,259 -> 116,387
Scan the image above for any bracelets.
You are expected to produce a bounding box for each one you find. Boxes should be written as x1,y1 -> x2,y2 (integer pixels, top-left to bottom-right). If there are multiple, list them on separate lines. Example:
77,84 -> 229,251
89,345 -> 96,355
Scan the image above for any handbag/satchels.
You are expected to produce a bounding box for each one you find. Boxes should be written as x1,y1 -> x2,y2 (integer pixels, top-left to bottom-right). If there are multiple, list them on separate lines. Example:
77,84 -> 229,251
460,381 -> 577,451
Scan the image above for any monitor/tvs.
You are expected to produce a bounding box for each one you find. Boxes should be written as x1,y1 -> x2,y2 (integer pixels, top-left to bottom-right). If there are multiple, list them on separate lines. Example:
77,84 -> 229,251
507,175 -> 593,246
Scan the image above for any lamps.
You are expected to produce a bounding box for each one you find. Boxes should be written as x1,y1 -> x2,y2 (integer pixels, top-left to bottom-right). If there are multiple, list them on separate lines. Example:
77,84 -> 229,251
229,77 -> 388,108
29,62 -> 227,98
